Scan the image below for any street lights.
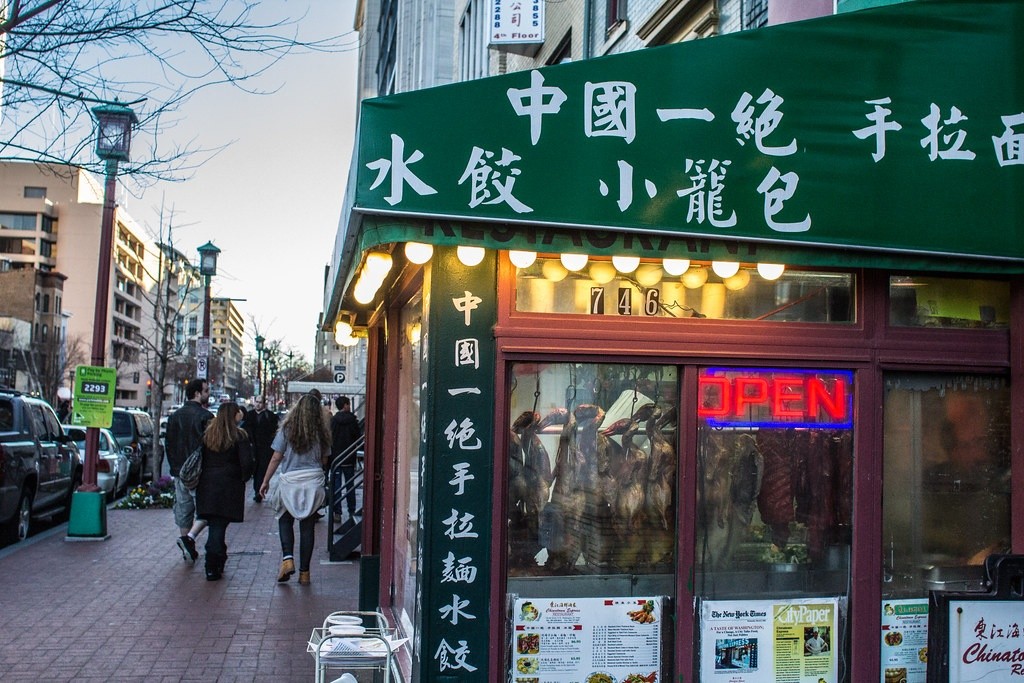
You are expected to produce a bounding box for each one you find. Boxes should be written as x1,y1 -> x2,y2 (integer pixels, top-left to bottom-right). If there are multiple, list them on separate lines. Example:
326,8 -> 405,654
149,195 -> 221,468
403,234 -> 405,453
75,94 -> 141,494
254,335 -> 266,398
261,346 -> 271,409
195,239 -> 222,407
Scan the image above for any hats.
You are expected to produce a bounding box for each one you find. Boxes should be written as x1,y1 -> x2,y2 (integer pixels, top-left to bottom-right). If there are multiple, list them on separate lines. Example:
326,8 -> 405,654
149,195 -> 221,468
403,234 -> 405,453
813,627 -> 818,633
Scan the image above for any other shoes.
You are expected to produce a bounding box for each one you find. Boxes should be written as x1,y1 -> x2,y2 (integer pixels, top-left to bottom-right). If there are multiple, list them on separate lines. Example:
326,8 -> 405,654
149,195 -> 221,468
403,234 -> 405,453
253,490 -> 263,502
333,513 -> 341,523
349,512 -> 356,518
204,566 -> 223,581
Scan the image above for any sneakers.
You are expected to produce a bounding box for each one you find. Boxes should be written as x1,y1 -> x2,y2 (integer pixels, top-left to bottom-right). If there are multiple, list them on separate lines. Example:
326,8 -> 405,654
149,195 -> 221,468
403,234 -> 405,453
298,571 -> 311,586
177,535 -> 198,566
278,559 -> 295,582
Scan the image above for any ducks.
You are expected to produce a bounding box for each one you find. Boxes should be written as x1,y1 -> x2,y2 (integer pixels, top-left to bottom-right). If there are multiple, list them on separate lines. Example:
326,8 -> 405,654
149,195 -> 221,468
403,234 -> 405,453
508,403 -> 733,572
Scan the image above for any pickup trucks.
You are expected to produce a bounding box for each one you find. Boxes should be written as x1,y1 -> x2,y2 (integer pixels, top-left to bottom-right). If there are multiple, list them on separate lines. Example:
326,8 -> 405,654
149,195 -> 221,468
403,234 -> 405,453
0,388 -> 84,546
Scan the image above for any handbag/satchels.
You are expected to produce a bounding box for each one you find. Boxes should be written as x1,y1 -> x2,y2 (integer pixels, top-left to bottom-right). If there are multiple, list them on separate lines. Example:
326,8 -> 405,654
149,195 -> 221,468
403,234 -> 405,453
179,446 -> 202,489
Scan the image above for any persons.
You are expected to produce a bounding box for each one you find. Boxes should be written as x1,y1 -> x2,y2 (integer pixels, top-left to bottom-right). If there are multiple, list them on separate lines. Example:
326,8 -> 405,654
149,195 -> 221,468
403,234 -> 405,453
125,406 -> 150,416
259,394 -> 330,586
804,627 -> 828,655
328,397 -> 364,522
165,378 -> 211,561
196,402 -> 253,580
303,388 -> 334,520
240,395 -> 278,502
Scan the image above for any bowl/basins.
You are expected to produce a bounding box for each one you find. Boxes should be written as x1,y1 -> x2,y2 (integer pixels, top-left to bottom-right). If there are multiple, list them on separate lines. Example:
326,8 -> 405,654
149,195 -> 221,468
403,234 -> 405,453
328,615 -> 365,649
330,673 -> 358,683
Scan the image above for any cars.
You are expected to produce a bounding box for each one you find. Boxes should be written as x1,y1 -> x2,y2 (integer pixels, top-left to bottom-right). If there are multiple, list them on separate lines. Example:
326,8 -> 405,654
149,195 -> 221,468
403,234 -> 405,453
57,401 -> 184,503
